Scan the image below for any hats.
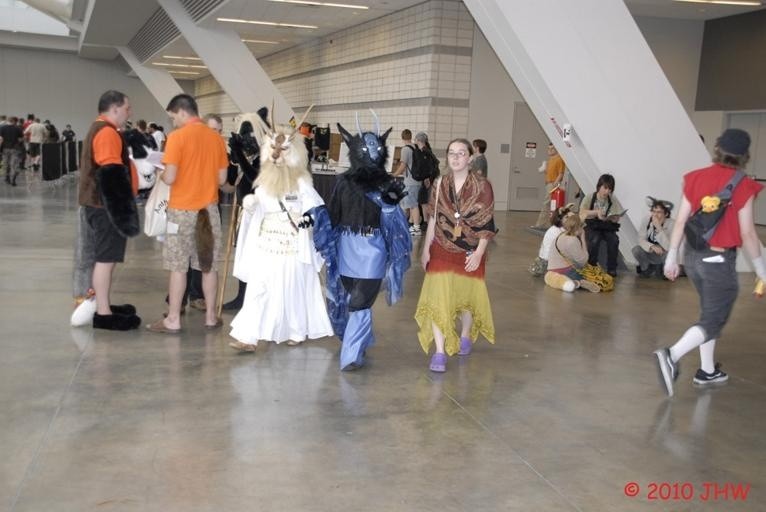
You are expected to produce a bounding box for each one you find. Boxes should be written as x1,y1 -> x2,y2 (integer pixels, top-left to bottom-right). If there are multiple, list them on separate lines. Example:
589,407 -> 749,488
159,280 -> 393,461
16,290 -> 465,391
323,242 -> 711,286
715,128 -> 751,155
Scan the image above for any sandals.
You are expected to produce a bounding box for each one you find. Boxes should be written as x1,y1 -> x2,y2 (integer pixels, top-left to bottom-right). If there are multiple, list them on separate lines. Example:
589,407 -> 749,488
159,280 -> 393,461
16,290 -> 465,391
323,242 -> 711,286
457,336 -> 472,357
429,352 -> 448,373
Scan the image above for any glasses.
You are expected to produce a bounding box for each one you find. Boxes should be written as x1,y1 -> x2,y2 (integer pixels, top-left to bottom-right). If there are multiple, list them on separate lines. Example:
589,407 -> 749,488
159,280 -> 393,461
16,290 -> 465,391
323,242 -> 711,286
447,152 -> 469,158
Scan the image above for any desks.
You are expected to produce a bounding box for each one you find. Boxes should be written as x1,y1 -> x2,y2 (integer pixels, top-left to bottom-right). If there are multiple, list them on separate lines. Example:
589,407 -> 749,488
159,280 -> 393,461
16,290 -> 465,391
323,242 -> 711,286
312,164 -> 405,202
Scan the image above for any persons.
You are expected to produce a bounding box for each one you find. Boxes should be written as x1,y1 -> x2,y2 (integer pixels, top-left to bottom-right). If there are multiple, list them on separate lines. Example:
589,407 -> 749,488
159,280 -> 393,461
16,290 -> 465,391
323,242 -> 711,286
0,91 -> 681,372
653,128 -> 765,396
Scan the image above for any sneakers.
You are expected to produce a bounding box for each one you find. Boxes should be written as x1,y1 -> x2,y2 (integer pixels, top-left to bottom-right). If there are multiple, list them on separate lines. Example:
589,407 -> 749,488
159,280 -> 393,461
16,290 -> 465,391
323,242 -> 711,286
653,346 -> 679,400
229,341 -> 257,353
606,264 -> 618,279
408,220 -> 429,237
578,278 -> 602,294
694,363 -> 729,387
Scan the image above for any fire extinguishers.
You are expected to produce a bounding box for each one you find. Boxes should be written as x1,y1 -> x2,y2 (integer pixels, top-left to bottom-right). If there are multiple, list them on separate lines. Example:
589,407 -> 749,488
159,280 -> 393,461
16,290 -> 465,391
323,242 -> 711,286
548,182 -> 566,223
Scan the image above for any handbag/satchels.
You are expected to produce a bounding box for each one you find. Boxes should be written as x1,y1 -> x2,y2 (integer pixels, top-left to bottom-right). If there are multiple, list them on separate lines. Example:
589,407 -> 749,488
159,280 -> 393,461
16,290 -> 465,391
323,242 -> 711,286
528,255 -> 547,278
142,171 -> 170,238
684,170 -> 746,251
584,219 -> 621,233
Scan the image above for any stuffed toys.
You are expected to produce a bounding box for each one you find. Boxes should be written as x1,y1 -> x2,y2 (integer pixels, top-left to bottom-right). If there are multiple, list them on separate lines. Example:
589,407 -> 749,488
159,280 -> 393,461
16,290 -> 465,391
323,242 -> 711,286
701,196 -> 720,213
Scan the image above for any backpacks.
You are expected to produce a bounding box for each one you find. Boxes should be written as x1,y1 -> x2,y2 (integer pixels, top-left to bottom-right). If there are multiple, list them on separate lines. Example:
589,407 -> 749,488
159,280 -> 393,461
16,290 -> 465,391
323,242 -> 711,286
402,143 -> 441,181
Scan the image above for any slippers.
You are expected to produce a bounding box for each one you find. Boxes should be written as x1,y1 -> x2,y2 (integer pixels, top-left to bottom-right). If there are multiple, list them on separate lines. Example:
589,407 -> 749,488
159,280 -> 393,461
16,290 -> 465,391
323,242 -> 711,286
146,318 -> 182,334
189,296 -> 224,331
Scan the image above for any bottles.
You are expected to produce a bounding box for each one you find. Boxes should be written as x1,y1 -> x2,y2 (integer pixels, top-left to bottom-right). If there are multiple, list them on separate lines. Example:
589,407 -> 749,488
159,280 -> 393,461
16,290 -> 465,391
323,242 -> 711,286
752,279 -> 766,299
